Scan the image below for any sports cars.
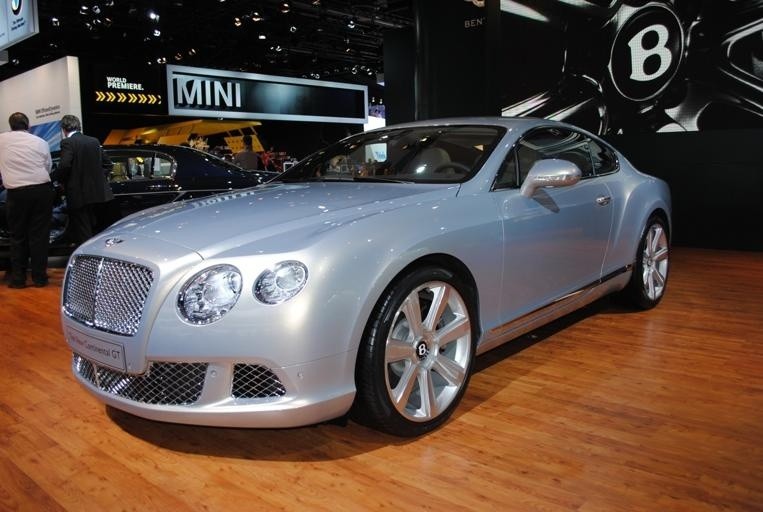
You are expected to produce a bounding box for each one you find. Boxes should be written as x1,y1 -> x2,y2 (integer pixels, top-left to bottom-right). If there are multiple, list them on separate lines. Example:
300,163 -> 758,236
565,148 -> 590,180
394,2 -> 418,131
60,115 -> 672,437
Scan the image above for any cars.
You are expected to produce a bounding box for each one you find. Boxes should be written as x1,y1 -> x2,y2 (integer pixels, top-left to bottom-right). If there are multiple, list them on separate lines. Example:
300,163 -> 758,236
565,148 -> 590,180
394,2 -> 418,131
0,144 -> 281,271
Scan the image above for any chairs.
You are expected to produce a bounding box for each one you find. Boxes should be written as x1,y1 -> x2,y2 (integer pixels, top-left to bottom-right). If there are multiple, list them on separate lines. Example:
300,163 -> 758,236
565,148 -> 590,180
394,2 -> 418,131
403,146 -> 455,173
500,146 -> 542,187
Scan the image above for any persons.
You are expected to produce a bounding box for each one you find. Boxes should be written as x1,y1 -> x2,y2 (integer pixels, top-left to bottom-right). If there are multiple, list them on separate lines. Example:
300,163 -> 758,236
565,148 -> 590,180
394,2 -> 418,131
56,114 -> 114,257
124,157 -> 141,179
0,112 -> 53,288
232,135 -> 265,171
